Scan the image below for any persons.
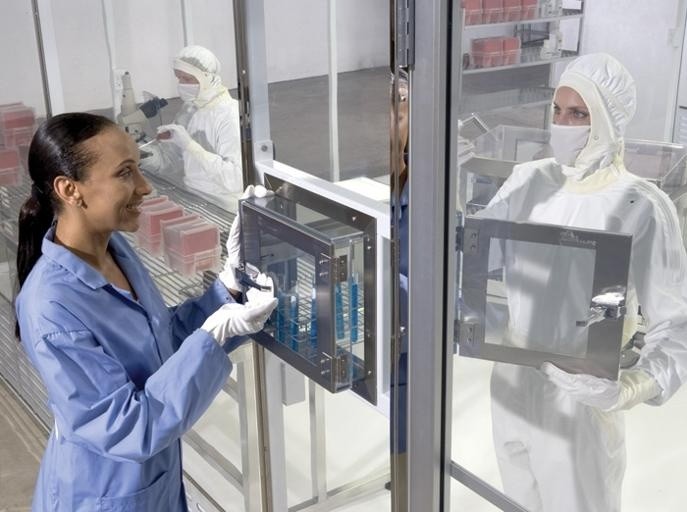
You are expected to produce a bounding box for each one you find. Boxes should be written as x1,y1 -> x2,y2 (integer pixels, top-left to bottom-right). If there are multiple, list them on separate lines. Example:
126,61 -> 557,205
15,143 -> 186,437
464,52 -> 683,512
131,46 -> 243,197
390,74 -> 415,453
14,111 -> 278,511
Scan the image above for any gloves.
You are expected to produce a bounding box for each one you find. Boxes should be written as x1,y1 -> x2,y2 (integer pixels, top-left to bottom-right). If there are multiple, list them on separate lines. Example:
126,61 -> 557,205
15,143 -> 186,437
200,272 -> 279,348
156,122 -> 193,154
216,184 -> 277,291
537,362 -> 623,413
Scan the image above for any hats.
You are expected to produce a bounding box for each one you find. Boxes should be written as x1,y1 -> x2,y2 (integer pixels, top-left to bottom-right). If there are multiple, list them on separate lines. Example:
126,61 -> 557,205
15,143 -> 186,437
553,52 -> 637,168
172,45 -> 221,91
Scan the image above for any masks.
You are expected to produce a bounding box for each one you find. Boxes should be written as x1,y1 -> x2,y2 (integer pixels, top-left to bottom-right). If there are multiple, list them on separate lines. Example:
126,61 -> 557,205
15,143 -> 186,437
548,123 -> 589,168
175,83 -> 200,103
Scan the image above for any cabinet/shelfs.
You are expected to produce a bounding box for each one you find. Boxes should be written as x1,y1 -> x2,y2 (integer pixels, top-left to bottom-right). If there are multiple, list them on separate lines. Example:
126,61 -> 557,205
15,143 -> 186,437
460,1 -> 585,143
